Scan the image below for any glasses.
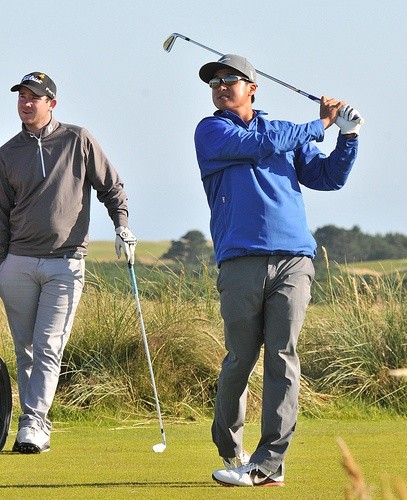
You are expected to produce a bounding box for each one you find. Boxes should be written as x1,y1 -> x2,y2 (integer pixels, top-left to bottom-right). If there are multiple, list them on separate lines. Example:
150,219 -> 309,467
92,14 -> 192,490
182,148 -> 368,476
209,75 -> 252,89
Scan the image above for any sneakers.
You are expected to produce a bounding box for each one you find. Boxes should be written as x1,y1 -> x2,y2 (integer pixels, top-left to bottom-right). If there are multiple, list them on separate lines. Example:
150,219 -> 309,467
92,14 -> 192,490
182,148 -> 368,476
212,452 -> 286,487
13,427 -> 51,454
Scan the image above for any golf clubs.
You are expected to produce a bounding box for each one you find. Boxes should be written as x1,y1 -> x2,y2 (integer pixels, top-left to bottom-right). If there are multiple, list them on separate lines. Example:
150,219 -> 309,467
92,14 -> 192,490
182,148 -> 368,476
125,251 -> 168,453
163,31 -> 358,121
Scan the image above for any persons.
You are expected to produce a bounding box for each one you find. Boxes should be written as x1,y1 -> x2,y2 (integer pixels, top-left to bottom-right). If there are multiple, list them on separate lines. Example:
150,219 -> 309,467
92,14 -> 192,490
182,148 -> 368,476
194,53 -> 360,488
0,72 -> 138,454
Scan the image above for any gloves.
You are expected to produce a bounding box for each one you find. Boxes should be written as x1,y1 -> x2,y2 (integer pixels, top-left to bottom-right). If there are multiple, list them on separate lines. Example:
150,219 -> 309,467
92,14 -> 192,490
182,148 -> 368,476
114,226 -> 136,265
334,102 -> 363,136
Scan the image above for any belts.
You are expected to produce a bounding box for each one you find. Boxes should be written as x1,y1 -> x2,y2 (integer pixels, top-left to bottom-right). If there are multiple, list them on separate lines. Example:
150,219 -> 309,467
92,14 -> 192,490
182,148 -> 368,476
54,253 -> 84,260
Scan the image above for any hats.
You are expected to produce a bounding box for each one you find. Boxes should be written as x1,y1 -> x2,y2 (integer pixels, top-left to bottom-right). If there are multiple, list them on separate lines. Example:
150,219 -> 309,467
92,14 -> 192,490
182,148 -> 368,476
199,54 -> 257,84
10,72 -> 56,100
